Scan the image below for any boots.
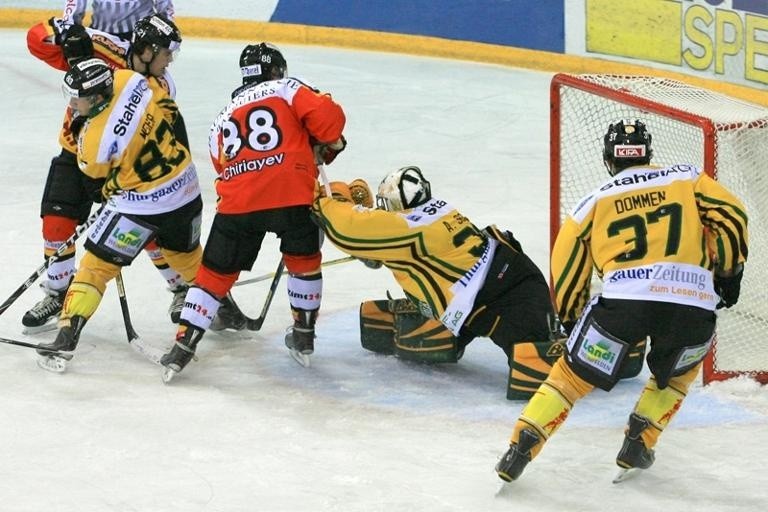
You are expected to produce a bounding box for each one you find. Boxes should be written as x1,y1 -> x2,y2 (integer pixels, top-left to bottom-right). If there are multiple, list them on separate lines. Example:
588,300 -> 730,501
496,430 -> 540,482
37,315 -> 87,360
617,414 -> 655,469
22,275 -> 74,326
284,310 -> 314,354
160,326 -> 202,372
168,284 -> 230,330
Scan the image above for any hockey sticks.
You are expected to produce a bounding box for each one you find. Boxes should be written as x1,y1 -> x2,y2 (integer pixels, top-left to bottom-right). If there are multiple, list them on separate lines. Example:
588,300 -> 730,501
102,192 -> 170,366
246,258 -> 287,332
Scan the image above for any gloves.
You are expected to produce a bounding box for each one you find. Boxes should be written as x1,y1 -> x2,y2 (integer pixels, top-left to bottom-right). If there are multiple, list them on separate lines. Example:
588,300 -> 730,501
714,262 -> 744,309
310,134 -> 346,165
61,25 -> 94,65
81,174 -> 107,202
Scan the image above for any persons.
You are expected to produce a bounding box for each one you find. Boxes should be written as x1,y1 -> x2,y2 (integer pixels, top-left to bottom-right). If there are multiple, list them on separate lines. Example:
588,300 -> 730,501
36,57 -> 247,361
310,159 -> 647,403
60,0 -> 175,35
493,117 -> 747,483
158,40 -> 347,372
21,14 -> 181,328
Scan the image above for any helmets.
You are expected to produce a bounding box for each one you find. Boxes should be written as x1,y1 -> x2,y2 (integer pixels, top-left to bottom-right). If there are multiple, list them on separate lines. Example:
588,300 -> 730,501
130,13 -> 181,55
375,166 -> 431,211
61,58 -> 113,110
603,119 -> 653,177
239,42 -> 287,83
